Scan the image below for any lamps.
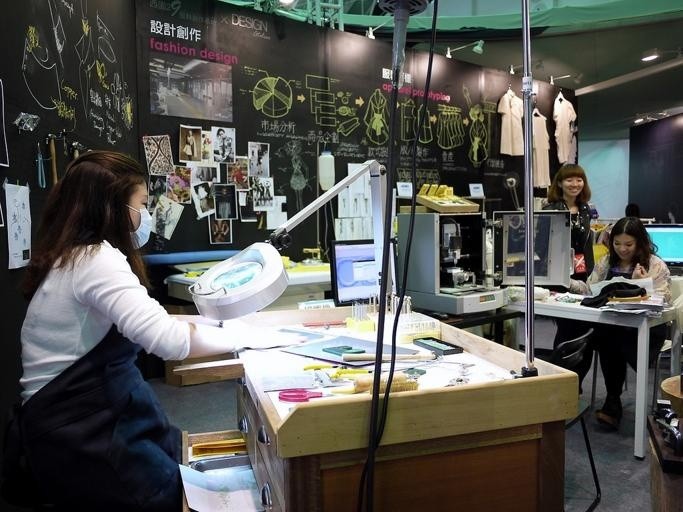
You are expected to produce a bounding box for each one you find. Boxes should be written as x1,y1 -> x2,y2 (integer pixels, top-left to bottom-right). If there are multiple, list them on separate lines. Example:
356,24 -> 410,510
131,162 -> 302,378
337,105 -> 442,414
554,73 -> 584,84
514,59 -> 545,72
640,47 -> 683,62
633,111 -> 672,124
450,39 -> 484,55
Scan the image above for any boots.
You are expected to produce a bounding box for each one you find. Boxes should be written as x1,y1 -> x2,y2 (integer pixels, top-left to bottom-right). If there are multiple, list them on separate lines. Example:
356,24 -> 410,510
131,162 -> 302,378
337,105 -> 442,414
595,394 -> 623,428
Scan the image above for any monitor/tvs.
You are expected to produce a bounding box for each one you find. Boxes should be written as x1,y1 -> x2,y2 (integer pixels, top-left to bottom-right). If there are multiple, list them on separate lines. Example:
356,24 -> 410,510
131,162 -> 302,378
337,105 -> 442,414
329,238 -> 401,307
642,223 -> 683,267
493,210 -> 572,293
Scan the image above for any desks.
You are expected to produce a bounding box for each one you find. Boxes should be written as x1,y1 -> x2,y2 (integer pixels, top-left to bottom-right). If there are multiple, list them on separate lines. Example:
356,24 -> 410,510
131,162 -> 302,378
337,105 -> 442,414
504,294 -> 683,460
173,303 -> 579,512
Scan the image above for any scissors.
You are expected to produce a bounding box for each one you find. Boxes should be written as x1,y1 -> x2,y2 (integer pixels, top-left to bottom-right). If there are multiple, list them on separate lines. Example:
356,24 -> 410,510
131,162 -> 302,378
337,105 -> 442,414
279,388 -> 336,402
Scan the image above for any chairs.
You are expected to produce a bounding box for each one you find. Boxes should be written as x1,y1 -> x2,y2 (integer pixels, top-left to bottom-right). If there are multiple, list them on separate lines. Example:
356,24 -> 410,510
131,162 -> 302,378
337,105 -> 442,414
591,275 -> 683,412
544,328 -> 601,512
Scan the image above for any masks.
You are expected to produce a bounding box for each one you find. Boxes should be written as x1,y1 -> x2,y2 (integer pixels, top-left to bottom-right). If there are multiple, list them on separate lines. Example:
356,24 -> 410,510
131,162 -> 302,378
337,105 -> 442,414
127,205 -> 152,249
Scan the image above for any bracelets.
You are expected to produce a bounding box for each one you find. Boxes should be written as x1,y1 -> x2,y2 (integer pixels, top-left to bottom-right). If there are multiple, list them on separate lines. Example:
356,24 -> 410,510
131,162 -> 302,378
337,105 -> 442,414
216,319 -> 223,328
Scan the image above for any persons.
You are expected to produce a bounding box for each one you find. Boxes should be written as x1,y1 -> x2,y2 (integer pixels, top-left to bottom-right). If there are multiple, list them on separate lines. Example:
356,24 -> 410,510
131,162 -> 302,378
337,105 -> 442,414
182,131 -> 195,161
568,215 -> 671,431
18,151 -> 307,512
194,183 -> 214,213
211,219 -> 229,242
214,129 -> 234,162
534,164 -> 594,398
625,203 -> 641,220
